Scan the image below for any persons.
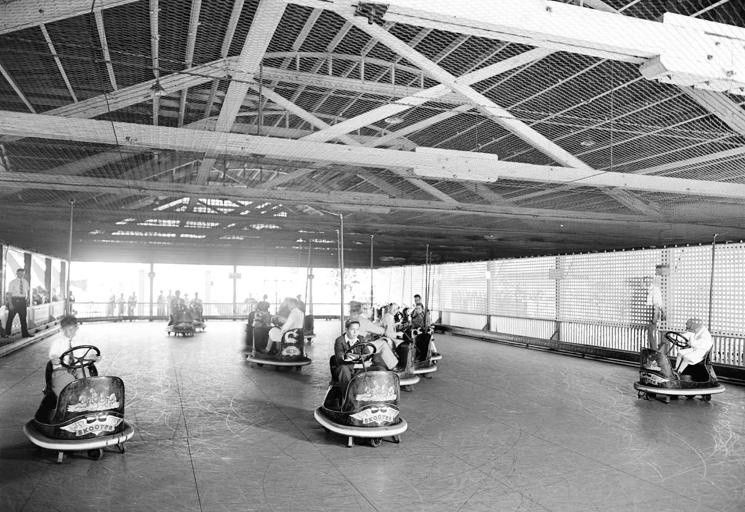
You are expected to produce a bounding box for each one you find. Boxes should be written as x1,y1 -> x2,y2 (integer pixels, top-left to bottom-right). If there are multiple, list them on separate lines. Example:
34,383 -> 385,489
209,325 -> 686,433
105,289 -> 204,334
671,318 -> 715,378
349,294 -> 433,373
244,291 -> 305,355
639,276 -> 668,349
48,314 -> 102,403
1,285 -> 78,319
5,268 -> 35,339
333,318 -> 372,407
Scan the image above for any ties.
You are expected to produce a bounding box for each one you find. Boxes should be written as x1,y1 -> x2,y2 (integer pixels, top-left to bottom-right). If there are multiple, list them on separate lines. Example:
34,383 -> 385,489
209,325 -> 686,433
20,279 -> 22,294
69,340 -> 74,366
